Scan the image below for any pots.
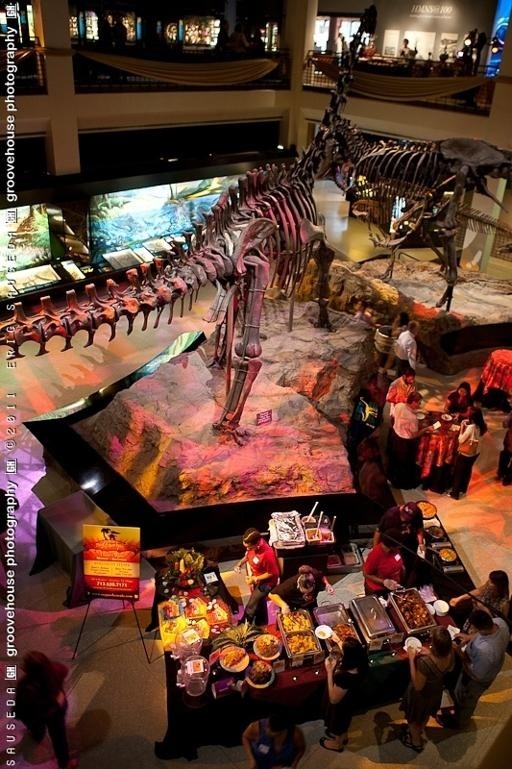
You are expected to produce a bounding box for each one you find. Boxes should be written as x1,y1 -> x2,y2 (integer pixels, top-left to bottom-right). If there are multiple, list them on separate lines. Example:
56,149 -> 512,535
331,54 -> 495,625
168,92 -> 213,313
416,500 -> 437,520
428,526 -> 445,541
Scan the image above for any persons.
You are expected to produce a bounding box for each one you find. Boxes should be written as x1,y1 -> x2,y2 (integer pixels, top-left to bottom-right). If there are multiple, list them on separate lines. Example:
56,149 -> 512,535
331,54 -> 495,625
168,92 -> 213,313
384,312 -> 409,369
448,570 -> 510,634
385,367 -> 416,456
397,627 -> 457,753
395,320 -> 419,378
358,436 -> 398,507
361,528 -> 407,596
242,716 -> 306,769
494,411 -> 512,486
267,565 -> 333,615
346,295 -> 362,315
398,38 -> 413,57
444,381 -> 475,417
232,527 -> 280,626
437,608 -> 510,731
313,33 -> 357,53
213,19 -> 265,54
449,406 -> 488,500
373,501 -> 426,584
318,632 -> 364,752
355,297 -> 382,328
10,649 -> 79,769
392,390 -> 436,490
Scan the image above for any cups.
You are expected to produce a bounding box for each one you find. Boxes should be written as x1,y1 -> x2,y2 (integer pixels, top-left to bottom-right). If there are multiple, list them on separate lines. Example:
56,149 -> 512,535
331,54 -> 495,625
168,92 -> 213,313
176,655 -> 210,696
169,629 -> 203,664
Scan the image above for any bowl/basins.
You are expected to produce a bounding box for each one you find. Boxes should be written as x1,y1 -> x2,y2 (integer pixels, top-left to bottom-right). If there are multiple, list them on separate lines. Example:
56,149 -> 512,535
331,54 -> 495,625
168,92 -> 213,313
349,595 -> 396,652
425,603 -> 436,616
313,600 -> 363,658
278,611 -> 322,668
434,600 -> 449,616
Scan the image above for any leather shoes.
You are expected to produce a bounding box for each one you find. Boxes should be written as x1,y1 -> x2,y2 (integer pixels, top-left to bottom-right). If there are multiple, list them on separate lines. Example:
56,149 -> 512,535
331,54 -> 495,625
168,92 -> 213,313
320,737 -> 344,752
325,728 -> 348,745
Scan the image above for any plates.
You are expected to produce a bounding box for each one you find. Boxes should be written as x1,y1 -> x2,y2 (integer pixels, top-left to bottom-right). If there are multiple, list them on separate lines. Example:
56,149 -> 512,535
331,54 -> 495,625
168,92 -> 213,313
388,588 -> 438,636
211,624 -> 238,634
220,647 -> 249,672
245,661 -> 274,688
441,414 -> 453,420
253,634 -> 283,658
316,625 -> 331,639
405,637 -> 422,654
439,546 -> 458,563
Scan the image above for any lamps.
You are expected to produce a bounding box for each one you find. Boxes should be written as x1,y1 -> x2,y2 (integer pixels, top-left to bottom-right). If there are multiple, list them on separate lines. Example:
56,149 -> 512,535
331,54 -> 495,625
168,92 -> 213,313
152,156 -> 185,175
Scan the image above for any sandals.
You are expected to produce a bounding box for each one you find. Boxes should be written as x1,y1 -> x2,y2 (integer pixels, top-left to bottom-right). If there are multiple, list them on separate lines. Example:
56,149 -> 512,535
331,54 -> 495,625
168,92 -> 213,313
400,733 -> 422,753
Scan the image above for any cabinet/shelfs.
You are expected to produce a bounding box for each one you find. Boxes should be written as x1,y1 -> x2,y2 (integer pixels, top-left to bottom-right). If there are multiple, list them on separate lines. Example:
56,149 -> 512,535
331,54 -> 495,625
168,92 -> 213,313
0,230 -> 197,316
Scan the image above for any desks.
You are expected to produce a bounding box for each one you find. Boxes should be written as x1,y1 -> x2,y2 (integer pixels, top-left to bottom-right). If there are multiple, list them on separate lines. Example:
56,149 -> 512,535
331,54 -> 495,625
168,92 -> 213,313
28,489 -> 119,609
267,511 -> 337,585
